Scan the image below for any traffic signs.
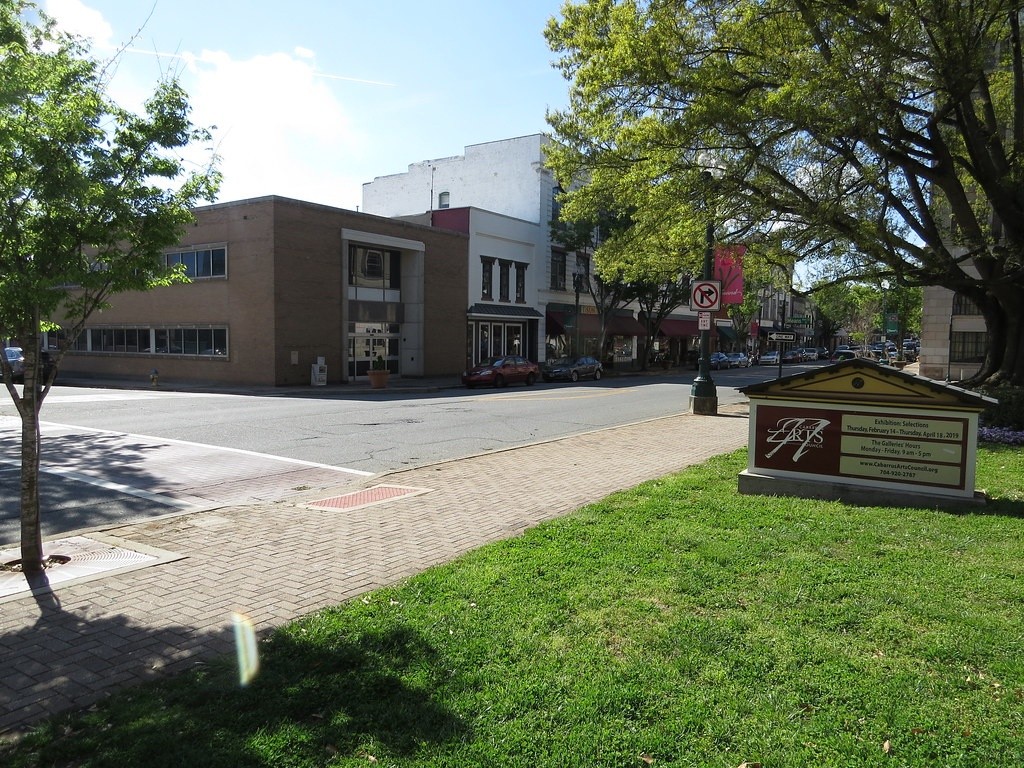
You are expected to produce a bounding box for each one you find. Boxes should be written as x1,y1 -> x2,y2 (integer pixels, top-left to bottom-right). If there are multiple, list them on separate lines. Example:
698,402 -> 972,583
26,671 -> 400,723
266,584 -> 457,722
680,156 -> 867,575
767,331 -> 796,343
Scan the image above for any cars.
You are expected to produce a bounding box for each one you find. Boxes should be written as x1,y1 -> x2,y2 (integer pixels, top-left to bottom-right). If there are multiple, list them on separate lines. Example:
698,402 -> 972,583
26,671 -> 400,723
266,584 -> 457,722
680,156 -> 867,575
698,352 -> 731,371
461,355 -> 539,389
758,351 -> 779,366
144,345 -> 182,353
541,355 -> 603,383
782,351 -> 802,363
831,338 -> 920,361
727,353 -> 750,369
795,347 -> 829,362
0,347 -> 57,386
200,348 -> 226,355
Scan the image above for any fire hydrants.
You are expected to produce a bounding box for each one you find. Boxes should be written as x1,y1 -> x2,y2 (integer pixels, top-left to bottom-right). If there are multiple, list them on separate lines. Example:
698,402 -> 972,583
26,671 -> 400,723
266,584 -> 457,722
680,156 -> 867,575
149,368 -> 159,388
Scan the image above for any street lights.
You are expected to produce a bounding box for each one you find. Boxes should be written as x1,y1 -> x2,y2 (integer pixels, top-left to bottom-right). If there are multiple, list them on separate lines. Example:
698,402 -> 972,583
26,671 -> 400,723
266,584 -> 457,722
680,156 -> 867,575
571,261 -> 586,355
688,152 -> 728,415
881,279 -> 890,359
